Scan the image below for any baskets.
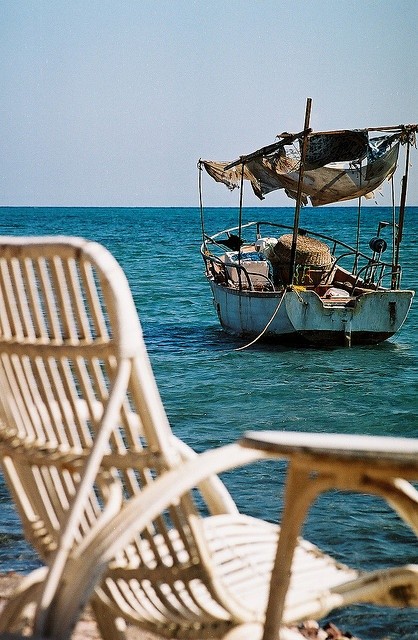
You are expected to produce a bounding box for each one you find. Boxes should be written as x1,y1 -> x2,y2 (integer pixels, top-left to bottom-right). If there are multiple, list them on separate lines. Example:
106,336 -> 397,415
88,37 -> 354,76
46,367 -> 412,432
273,258 -> 338,286
274,234 -> 332,267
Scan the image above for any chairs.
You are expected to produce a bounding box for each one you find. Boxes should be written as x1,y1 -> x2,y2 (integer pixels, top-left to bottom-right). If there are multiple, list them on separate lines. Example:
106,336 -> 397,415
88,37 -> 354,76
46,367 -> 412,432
0,234 -> 363,640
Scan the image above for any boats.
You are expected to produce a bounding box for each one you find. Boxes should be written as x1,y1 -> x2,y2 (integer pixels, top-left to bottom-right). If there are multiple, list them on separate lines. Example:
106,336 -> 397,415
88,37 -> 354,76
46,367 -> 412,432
201,221 -> 414,345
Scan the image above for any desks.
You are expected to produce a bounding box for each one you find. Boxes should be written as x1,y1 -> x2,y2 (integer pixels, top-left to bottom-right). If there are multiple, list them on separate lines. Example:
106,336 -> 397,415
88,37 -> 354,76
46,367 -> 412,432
241,430 -> 418,640
